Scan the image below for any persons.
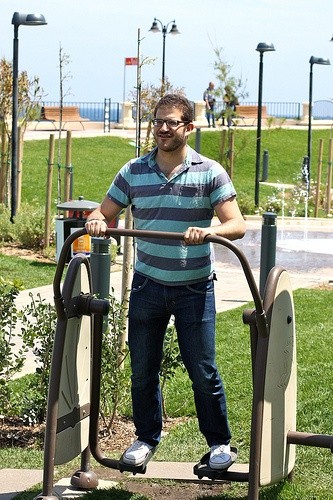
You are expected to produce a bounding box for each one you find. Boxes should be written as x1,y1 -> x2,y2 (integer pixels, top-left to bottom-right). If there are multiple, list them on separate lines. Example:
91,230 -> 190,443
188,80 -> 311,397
85,94 -> 247,469
204,82 -> 217,128
214,85 -> 239,127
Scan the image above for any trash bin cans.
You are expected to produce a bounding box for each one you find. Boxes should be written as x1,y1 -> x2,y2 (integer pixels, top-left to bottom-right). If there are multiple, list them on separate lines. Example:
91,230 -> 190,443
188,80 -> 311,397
55,196 -> 106,266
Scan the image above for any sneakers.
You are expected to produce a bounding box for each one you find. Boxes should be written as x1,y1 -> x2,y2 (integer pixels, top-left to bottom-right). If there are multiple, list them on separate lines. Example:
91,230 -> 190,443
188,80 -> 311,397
123,440 -> 153,465
209,443 -> 232,469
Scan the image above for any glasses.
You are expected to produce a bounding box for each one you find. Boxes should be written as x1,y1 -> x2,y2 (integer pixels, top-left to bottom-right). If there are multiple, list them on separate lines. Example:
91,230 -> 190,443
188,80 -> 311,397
151,118 -> 191,127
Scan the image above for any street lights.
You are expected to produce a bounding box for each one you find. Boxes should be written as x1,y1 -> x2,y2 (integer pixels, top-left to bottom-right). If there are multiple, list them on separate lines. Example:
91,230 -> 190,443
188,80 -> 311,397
305,56 -> 332,194
9,11 -> 48,222
253,42 -> 275,208
149,18 -> 181,95
